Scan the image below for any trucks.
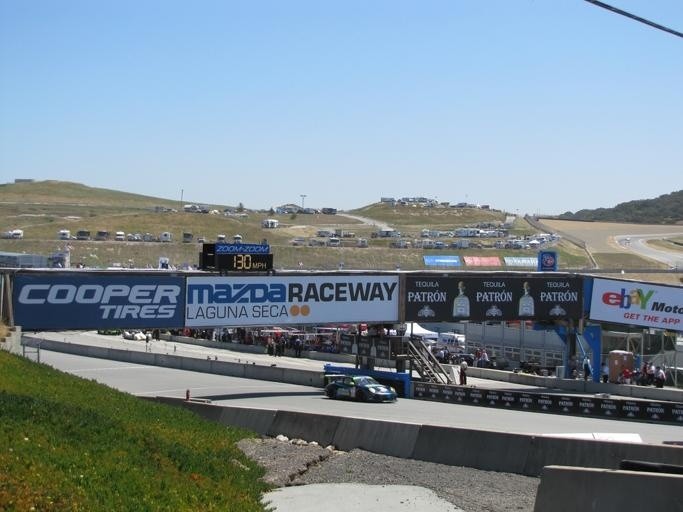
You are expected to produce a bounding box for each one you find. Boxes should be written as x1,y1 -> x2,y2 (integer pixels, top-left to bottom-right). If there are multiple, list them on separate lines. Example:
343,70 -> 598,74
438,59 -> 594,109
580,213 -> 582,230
436,332 -> 465,352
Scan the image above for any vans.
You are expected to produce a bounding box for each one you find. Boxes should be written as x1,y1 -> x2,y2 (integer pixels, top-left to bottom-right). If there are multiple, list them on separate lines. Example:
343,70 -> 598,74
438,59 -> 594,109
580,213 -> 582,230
12,229 -> 23,239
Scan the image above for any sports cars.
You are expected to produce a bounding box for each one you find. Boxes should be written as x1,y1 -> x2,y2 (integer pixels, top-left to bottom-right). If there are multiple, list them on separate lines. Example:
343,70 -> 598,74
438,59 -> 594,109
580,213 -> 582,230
320,373 -> 398,402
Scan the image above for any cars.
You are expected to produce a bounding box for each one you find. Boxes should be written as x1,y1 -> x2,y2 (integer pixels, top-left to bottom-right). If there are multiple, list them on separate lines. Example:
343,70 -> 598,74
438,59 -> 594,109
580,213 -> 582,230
95,324 -> 368,346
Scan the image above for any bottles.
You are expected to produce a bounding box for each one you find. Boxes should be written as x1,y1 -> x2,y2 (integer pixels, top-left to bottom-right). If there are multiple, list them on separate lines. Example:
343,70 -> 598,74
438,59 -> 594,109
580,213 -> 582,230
452,281 -> 471,317
518,281 -> 534,316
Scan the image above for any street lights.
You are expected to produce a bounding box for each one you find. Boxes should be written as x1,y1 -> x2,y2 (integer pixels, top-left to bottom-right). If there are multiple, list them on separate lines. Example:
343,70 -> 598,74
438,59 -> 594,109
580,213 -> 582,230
180,188 -> 183,209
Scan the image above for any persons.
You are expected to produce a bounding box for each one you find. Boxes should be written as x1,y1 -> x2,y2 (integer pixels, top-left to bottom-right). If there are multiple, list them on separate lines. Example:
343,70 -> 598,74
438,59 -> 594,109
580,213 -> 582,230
143,324 -> 301,358
459,357 -> 467,385
437,344 -> 488,366
572,357 -> 666,388
